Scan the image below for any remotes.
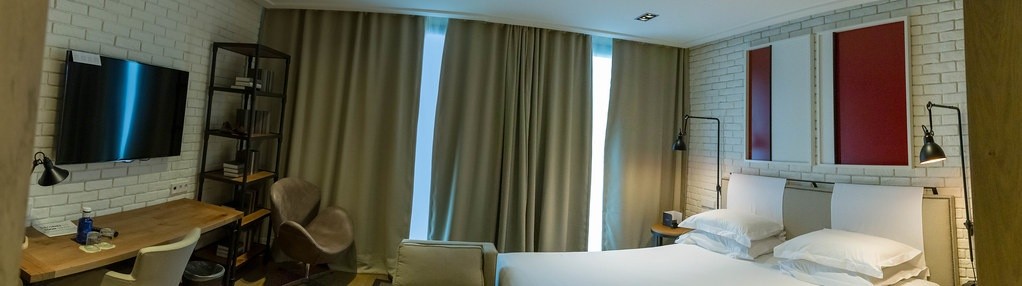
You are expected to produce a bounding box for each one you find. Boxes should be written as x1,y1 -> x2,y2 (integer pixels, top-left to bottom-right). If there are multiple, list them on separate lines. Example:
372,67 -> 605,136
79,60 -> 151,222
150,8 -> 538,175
91,226 -> 119,239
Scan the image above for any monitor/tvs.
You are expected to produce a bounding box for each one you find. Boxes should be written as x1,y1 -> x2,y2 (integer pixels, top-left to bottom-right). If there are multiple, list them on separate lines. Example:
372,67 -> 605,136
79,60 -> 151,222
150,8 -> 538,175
55,49 -> 190,165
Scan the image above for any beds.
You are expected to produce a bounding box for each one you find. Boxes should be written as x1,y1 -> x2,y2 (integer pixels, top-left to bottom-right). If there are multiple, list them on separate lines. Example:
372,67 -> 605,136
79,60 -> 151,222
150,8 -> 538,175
493,175 -> 960,286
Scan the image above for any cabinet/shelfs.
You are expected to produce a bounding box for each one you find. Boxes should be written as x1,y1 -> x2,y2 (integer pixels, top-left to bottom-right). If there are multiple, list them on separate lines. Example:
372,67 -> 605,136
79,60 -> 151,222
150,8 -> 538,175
195,41 -> 291,286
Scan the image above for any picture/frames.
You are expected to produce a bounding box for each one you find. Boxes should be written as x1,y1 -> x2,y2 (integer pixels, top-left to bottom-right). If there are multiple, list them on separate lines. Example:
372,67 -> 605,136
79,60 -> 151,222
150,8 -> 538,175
816,15 -> 916,167
744,33 -> 815,167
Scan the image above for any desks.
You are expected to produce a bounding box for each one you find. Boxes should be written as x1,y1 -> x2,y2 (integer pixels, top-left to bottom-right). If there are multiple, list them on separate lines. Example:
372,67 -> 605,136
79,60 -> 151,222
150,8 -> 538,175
15,197 -> 244,286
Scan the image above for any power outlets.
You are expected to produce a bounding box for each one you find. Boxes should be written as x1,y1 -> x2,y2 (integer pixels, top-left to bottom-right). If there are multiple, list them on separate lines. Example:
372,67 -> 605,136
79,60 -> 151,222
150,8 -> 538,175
170,181 -> 188,196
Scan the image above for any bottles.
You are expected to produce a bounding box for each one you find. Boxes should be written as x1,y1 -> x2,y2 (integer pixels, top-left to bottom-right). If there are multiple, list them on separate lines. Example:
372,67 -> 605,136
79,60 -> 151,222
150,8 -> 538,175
75,206 -> 94,244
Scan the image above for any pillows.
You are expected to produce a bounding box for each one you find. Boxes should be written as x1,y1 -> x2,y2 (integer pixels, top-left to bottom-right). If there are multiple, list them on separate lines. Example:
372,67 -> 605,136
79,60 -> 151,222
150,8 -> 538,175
675,229 -> 787,260
777,259 -> 931,286
773,228 -> 922,278
677,210 -> 785,248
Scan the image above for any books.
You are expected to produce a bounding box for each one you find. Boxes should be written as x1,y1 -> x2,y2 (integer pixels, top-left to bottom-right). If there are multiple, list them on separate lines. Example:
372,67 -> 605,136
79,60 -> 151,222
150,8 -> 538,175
32,220 -> 79,238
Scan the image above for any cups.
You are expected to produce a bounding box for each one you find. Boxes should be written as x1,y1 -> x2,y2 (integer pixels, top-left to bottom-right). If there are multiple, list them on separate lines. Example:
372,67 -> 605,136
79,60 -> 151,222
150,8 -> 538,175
99,227 -> 114,248
86,231 -> 101,251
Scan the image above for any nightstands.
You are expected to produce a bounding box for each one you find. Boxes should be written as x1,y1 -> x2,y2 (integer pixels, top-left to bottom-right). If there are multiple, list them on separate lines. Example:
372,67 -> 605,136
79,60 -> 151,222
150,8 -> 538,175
650,222 -> 695,247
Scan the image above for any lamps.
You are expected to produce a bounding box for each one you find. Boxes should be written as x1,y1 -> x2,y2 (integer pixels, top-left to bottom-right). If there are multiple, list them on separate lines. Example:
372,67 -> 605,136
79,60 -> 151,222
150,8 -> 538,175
672,114 -> 722,210
20,151 -> 70,250
920,101 -> 977,286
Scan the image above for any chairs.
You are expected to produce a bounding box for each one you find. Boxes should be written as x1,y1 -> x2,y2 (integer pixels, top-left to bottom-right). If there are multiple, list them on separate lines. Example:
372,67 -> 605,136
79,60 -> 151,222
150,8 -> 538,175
269,176 -> 356,286
98,227 -> 201,286
393,238 -> 498,286
